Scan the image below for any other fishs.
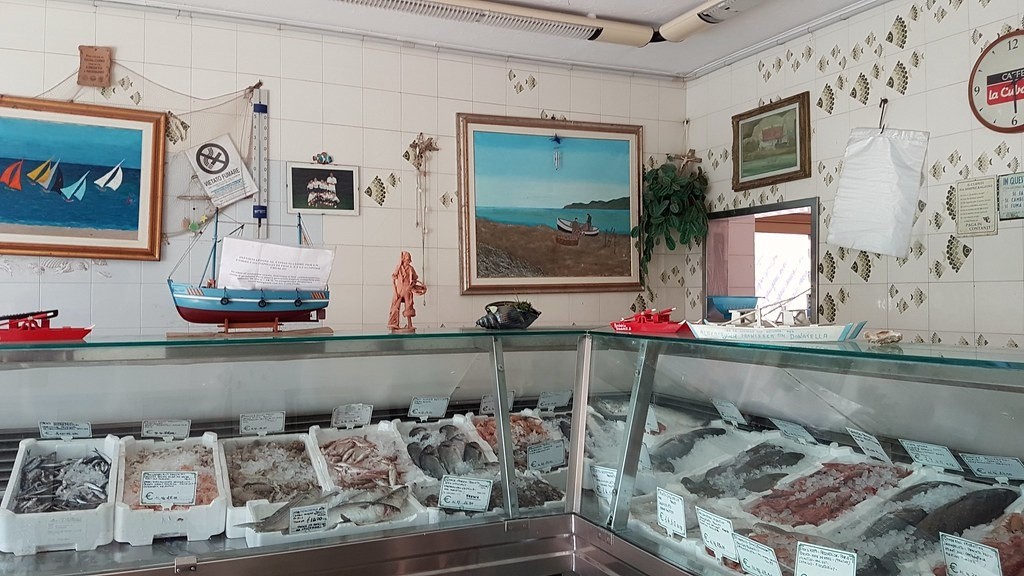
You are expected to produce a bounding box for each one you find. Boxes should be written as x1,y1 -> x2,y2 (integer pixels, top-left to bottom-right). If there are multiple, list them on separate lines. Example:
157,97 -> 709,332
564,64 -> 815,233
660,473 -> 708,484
9,420 -> 562,542
836,476 -> 1018,576
543,391 -> 805,503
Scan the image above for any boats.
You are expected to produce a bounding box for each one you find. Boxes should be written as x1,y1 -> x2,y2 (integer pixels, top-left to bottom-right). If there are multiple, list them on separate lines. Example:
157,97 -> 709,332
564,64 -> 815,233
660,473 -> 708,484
686,307 -> 868,344
611,307 -> 701,334
556,218 -> 600,237
167,206 -> 332,324
0,312 -> 94,345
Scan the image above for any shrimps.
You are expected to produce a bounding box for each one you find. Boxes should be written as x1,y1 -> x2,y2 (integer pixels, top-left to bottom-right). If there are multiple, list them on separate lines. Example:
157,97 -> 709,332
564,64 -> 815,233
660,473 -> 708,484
475,409 -> 547,451
749,459 -> 913,566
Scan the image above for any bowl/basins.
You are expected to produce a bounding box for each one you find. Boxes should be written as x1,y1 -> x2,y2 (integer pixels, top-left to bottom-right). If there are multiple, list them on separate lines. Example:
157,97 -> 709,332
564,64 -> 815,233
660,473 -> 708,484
712,296 -> 758,319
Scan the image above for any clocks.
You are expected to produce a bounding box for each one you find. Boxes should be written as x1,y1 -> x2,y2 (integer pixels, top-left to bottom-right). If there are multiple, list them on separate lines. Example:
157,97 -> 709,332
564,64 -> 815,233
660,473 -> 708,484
968,29 -> 1024,134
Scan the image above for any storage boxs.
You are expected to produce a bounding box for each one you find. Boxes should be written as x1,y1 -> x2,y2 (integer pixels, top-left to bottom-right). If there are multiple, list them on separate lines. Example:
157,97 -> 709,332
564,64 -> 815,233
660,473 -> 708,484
113,435 -> 228,548
532,407 -> 618,491
635,416 -> 765,495
0,432 -> 121,557
404,470 -> 567,524
810,466 -> 1023,548
305,420 -> 423,496
389,412 -> 497,482
217,430 -> 326,541
675,430 -> 852,511
462,406 -> 570,492
240,483 -> 430,548
0,323 -> 1023,576
625,487 -> 756,576
737,445 -> 929,537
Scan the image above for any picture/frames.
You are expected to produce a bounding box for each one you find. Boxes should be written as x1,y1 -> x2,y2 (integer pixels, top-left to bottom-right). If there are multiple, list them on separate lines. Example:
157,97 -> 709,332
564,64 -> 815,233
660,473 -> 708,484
731,91 -> 811,191
455,111 -> 646,295
0,93 -> 166,261
286,161 -> 360,216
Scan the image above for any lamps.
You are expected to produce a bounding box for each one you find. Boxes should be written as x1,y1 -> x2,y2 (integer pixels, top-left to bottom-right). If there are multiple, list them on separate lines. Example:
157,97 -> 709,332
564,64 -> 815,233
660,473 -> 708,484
334,1 -> 764,49
